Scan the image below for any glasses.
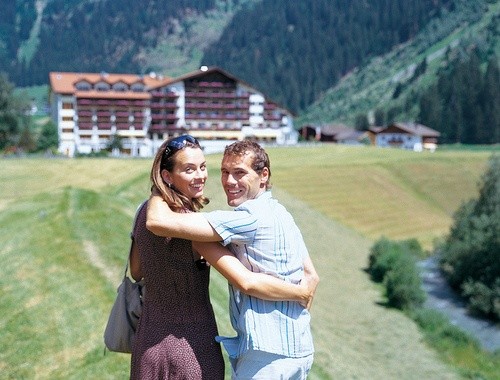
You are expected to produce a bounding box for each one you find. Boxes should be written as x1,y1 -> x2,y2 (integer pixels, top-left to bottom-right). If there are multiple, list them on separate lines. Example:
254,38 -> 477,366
162,135 -> 196,161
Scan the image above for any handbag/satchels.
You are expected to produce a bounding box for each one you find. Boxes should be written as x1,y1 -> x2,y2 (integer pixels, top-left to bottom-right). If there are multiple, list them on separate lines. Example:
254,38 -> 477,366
103,276 -> 143,354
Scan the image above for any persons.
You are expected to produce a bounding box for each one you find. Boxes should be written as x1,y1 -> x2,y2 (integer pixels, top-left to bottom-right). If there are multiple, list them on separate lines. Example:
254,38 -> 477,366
131,135 -> 311,380
146,142 -> 319,380
